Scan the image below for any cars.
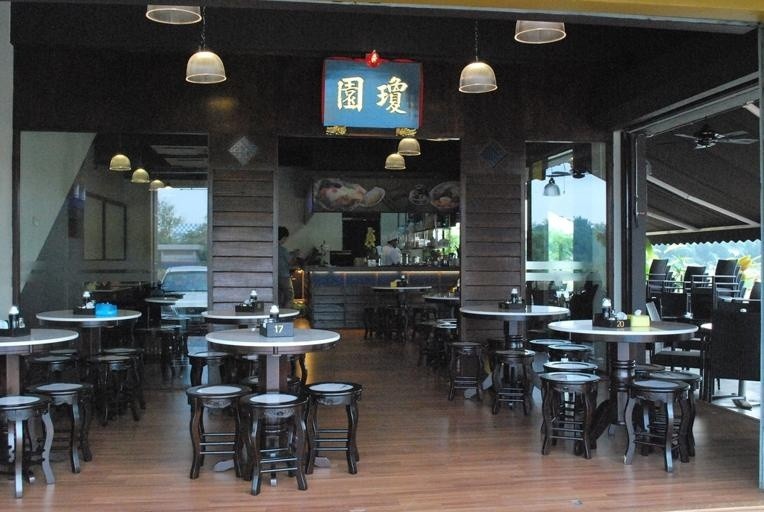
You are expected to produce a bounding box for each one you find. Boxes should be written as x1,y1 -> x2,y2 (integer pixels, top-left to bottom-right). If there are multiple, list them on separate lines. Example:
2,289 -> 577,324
160,264 -> 208,335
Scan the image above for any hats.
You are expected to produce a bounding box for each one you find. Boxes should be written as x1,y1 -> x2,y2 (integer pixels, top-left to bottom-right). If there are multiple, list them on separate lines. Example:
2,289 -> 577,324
388,233 -> 398,241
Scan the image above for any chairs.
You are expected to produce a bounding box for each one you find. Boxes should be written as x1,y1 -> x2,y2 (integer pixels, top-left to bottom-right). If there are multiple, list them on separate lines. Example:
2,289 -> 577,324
646,258 -> 761,410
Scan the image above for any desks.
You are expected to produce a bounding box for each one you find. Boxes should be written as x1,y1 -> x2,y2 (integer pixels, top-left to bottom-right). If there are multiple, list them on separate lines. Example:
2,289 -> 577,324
370,284 -> 461,336
33,280 -> 184,358
200,306 -> 300,376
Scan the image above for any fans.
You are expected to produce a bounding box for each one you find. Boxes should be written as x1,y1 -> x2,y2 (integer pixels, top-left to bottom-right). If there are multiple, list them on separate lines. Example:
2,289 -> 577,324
667,117 -> 759,151
546,159 -> 590,180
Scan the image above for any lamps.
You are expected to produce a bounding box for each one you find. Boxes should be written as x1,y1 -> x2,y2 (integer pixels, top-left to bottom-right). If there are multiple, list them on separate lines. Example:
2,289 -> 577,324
383,139 -> 422,173
543,174 -> 560,197
105,134 -> 165,193
144,4 -> 226,85
457,20 -> 496,95
513,19 -> 566,46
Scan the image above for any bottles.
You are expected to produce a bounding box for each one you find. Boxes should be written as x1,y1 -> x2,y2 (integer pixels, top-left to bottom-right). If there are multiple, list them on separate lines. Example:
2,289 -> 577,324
9,305 -> 25,328
244,288 -> 257,306
601,298 -> 615,321
511,288 -> 522,304
82,290 -> 96,308
263,304 -> 279,326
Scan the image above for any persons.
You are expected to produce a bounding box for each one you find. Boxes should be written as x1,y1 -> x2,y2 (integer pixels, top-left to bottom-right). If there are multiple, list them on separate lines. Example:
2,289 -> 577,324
277,226 -> 295,306
381,232 -> 401,265
327,181 -> 345,202
315,179 -> 348,212
584,269 -> 605,320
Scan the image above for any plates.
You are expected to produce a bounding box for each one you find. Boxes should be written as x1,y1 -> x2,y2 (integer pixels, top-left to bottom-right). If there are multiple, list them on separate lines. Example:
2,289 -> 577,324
429,181 -> 461,210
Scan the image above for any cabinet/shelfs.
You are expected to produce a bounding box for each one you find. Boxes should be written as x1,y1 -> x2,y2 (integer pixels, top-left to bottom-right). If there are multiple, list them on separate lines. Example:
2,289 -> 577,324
399,212 -> 450,265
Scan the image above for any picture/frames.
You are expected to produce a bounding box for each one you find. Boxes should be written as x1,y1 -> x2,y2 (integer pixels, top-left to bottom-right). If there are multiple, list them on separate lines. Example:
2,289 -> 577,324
82,192 -> 127,262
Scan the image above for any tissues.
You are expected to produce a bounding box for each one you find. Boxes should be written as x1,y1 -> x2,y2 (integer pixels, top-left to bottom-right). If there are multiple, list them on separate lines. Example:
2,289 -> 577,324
627,309 -> 650,326
390,279 -> 401,287
95,301 -> 118,316
